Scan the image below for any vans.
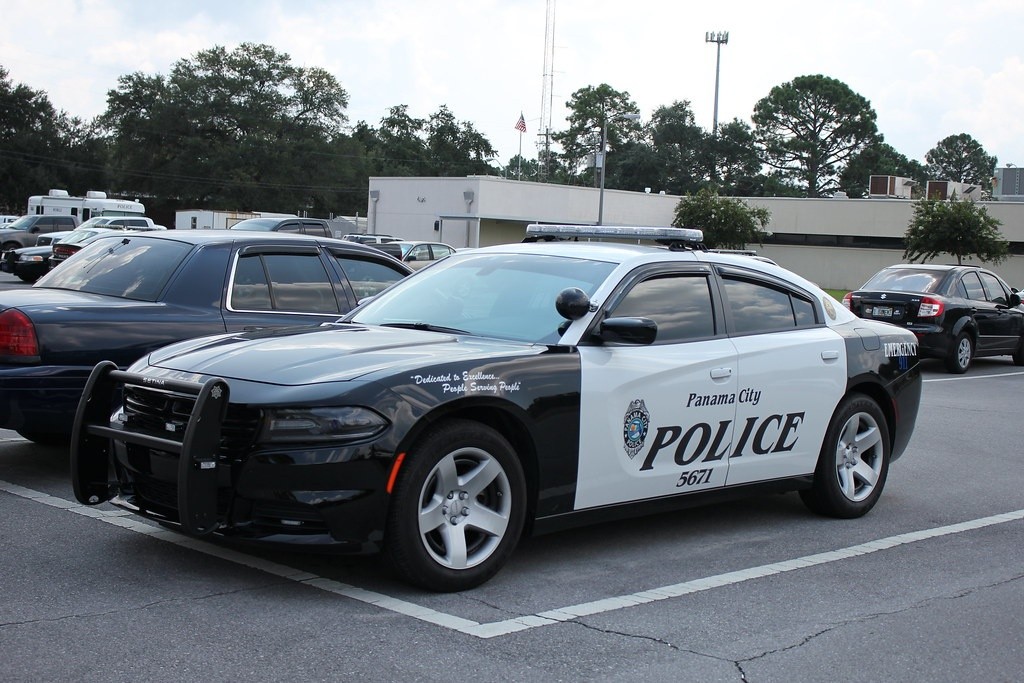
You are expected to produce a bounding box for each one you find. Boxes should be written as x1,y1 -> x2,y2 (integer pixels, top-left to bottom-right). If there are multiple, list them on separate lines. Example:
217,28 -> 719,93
27,189 -> 146,226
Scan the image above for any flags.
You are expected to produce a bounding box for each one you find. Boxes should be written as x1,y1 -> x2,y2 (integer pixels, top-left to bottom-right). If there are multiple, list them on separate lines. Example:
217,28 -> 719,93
515,115 -> 526,132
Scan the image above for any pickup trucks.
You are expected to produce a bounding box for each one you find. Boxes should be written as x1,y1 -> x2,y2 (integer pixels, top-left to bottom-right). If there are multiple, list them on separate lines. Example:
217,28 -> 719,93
0,213 -> 82,267
229,217 -> 333,238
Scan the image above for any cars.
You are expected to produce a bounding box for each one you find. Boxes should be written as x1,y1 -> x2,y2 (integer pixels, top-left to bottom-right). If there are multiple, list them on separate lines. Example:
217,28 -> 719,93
0,231 -> 419,470
0,217 -> 168,282
69,225 -> 923,594
343,232 -> 458,274
842,264 -> 1024,374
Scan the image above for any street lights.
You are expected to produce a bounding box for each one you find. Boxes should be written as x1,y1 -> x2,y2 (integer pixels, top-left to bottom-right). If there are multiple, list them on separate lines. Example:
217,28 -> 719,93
598,114 -> 639,224
1006,162 -> 1018,193
